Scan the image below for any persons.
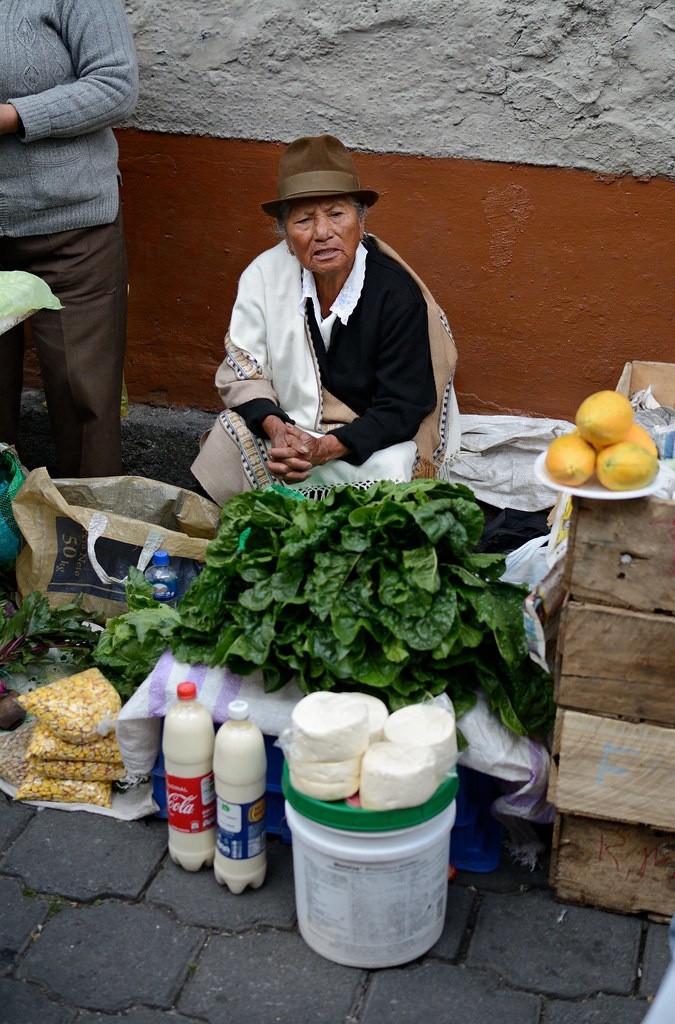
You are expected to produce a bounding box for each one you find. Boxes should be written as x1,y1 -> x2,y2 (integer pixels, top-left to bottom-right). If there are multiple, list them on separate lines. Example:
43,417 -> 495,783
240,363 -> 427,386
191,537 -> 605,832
0,0 -> 140,480
190,134 -> 462,509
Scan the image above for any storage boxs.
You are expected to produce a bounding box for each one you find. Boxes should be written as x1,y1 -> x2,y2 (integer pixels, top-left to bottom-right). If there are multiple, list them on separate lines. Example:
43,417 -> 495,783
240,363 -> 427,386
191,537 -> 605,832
548,814 -> 674,926
545,708 -> 675,832
553,595 -> 675,730
566,358 -> 674,617
151,723 -> 502,876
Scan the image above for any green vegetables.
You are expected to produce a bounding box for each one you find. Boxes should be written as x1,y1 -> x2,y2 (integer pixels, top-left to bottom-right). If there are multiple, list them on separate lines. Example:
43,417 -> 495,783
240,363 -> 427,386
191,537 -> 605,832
0,479 -> 551,740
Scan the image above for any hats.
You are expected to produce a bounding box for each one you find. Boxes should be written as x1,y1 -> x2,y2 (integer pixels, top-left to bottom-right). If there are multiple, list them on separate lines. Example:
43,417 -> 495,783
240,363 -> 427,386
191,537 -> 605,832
259,134 -> 379,219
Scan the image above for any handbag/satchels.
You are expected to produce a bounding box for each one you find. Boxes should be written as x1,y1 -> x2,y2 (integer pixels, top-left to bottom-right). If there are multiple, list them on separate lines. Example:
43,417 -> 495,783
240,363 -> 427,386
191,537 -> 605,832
11,465 -> 222,626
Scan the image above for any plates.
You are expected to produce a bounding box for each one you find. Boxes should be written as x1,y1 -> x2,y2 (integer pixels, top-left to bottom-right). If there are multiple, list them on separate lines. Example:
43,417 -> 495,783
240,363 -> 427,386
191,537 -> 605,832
534,450 -> 665,499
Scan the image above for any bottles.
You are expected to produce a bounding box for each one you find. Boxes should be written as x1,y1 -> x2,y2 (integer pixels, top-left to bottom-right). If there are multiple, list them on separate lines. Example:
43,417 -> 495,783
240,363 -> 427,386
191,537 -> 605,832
145,551 -> 177,608
213,700 -> 267,894
163,682 -> 216,871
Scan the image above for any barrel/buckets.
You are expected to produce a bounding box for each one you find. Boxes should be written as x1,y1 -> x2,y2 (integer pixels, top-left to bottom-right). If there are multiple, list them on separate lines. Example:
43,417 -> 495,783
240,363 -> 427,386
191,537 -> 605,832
281,757 -> 460,969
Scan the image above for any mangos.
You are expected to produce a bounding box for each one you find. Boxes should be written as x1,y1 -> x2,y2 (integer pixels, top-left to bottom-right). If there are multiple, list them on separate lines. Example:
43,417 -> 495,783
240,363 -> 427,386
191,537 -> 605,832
544,390 -> 661,490
